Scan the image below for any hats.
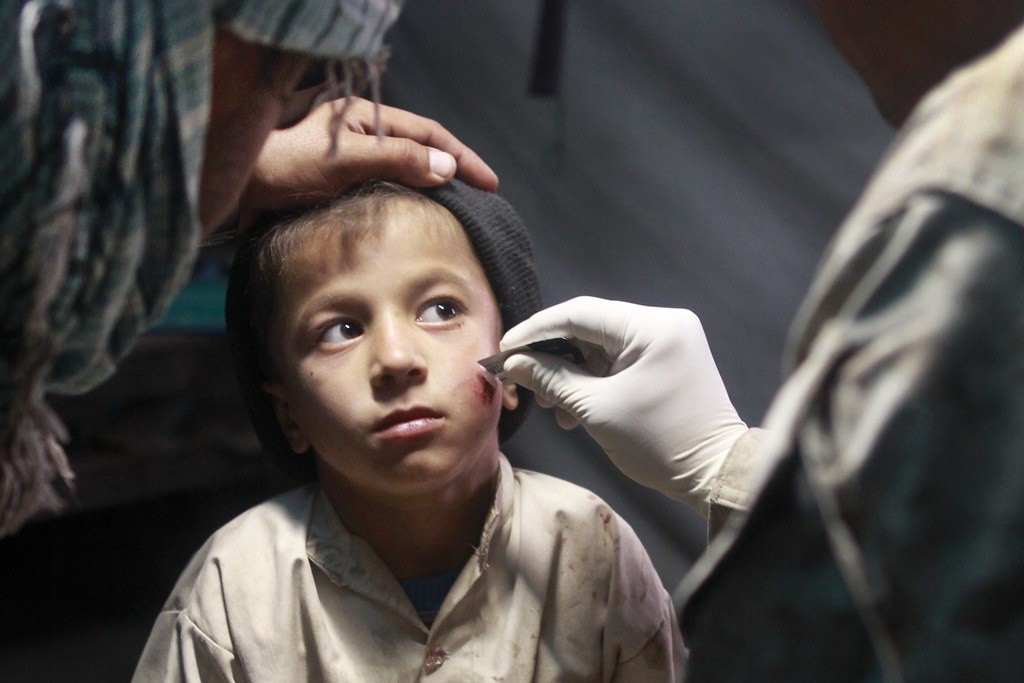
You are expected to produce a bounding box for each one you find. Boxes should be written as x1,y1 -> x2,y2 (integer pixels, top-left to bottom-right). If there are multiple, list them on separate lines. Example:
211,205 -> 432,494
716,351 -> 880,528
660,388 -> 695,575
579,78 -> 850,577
222,173 -> 544,440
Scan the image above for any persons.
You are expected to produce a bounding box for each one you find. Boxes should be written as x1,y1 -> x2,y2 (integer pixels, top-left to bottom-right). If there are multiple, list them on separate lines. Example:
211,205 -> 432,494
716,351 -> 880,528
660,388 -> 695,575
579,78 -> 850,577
1,1 -> 501,538
475,18 -> 1024,683
127,169 -> 692,683
